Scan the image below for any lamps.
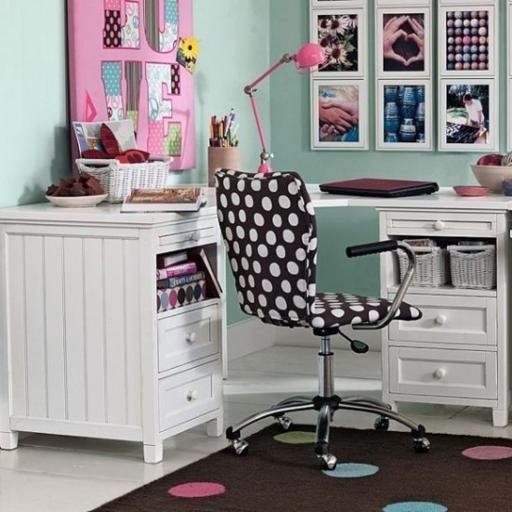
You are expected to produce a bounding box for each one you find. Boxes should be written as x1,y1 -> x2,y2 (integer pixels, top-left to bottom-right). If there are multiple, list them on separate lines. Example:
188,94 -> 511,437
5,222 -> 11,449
243,43 -> 331,174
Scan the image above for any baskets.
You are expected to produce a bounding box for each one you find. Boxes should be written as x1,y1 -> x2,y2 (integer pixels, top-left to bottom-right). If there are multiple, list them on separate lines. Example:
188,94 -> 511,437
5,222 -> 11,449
444,241 -> 499,292
74,154 -> 178,203
395,244 -> 454,286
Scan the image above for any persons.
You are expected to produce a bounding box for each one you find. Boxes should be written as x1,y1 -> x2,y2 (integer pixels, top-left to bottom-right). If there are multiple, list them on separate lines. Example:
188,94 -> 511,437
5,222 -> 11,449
462,94 -> 486,132
383,14 -> 425,67
319,98 -> 359,137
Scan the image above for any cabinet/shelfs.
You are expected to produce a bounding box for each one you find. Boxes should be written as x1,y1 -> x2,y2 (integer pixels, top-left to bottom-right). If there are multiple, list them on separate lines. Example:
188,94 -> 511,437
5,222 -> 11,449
379,208 -> 511,428
0,212 -> 227,464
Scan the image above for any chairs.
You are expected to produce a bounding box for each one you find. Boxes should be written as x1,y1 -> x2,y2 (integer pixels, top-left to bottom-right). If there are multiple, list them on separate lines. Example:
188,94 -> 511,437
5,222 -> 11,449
214,168 -> 430,471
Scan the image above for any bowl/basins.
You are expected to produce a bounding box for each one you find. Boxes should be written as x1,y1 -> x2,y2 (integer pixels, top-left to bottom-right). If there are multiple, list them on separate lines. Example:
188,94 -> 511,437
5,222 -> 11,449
470,165 -> 512,194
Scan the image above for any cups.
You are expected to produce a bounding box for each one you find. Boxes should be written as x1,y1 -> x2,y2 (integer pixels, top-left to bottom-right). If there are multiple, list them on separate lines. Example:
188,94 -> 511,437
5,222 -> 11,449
208,146 -> 241,187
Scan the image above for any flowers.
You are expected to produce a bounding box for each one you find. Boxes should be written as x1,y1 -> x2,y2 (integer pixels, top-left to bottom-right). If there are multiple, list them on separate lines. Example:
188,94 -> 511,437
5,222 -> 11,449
317,16 -> 358,71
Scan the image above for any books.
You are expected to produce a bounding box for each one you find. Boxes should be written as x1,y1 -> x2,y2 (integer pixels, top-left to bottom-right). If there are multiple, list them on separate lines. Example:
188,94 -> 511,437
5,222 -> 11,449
157,247 -> 222,313
122,187 -> 208,212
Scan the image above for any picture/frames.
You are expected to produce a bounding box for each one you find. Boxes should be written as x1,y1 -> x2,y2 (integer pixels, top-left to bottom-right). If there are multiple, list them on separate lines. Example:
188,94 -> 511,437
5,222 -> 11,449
438,78 -> 499,152
309,9 -> 368,80
375,7 -> 432,79
375,0 -> 432,7
438,0 -> 498,6
375,79 -> 433,152
309,0 -> 367,9
310,80 -> 369,151
438,5 -> 499,78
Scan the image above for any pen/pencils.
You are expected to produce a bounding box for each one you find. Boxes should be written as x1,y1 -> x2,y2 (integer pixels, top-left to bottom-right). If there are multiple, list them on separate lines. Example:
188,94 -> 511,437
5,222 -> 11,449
209,107 -> 239,148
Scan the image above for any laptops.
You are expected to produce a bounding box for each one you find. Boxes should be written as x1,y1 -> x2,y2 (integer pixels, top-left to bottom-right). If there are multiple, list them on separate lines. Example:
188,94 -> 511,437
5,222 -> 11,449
319,177 -> 439,197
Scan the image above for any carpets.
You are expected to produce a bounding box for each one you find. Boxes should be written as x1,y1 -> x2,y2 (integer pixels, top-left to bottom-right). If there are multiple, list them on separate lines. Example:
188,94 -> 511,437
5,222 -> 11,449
88,422 -> 512,512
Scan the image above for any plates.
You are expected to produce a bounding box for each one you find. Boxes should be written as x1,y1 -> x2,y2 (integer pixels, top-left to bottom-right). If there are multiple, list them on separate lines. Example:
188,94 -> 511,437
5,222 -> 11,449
454,185 -> 490,197
45,193 -> 109,208
